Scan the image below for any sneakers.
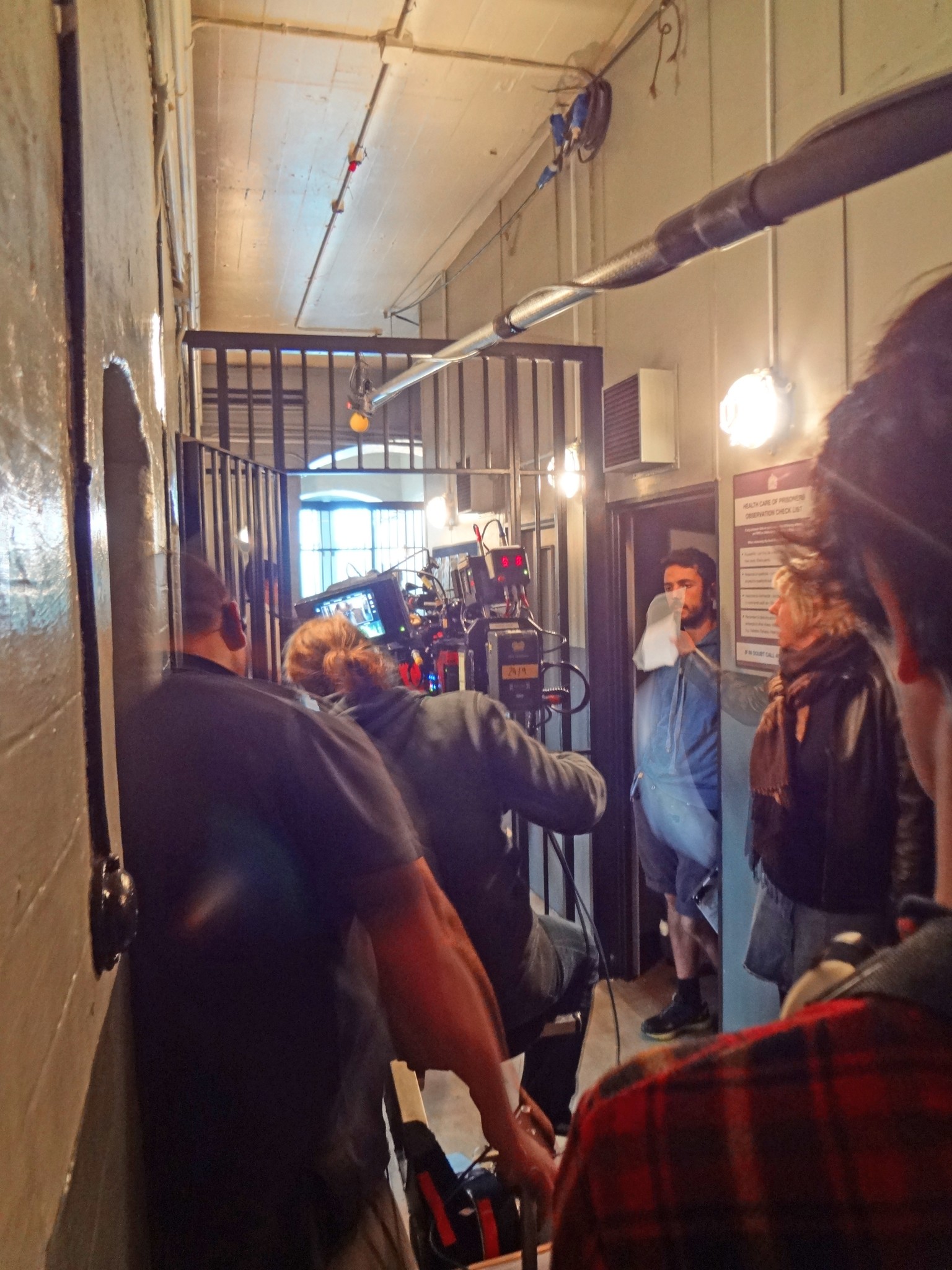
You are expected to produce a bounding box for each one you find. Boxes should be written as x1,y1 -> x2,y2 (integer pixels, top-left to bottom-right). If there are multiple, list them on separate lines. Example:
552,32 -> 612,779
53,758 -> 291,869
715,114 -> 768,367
640,997 -> 712,1041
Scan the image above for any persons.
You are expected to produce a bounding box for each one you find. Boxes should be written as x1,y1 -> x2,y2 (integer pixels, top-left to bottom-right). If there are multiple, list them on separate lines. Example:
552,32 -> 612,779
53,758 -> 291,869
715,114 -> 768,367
674,558 -> 935,1024
130,551 -> 606,1270
548,261 -> 951,1270
626,548 -> 723,1040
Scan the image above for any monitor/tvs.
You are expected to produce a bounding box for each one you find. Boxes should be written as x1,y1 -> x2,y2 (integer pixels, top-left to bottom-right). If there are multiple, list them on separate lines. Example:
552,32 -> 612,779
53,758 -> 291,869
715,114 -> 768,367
293,573 -> 416,653
